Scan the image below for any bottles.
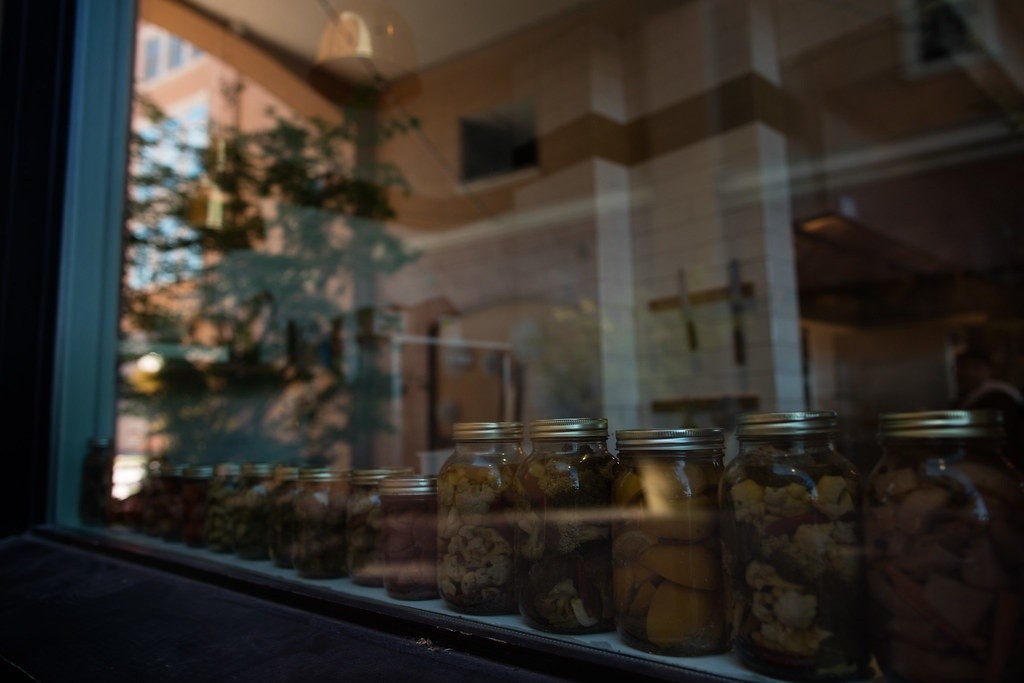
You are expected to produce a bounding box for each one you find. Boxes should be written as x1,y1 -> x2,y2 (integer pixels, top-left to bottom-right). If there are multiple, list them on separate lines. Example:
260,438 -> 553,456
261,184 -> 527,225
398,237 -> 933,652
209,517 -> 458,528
144,410 -> 1023,679
